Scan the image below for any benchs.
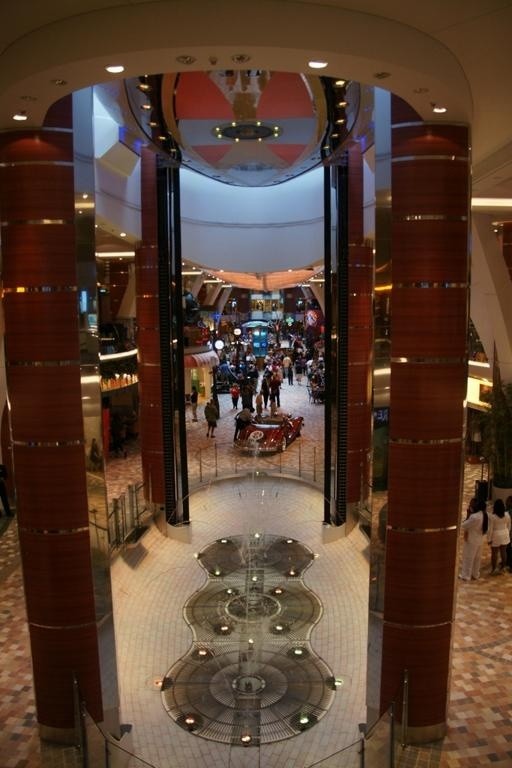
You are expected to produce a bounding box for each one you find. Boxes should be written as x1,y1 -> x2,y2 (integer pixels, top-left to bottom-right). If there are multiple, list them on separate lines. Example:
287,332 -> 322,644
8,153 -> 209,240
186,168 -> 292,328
260,416 -> 283,425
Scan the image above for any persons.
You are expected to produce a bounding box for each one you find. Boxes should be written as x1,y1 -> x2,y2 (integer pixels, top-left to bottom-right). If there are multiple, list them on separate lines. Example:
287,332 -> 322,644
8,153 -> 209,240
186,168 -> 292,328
188,296 -> 327,442
498,495 -> 511,573
0,463 -> 15,519
485,498 -> 511,577
456,496 -> 489,582
89,438 -> 102,467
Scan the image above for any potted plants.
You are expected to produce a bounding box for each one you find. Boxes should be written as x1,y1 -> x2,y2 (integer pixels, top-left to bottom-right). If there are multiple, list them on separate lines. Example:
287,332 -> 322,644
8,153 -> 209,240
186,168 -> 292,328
480,380 -> 512,510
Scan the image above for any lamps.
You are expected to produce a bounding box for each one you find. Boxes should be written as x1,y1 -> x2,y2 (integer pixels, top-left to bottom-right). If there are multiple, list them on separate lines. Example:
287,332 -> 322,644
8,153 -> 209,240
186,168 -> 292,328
233,294 -> 241,336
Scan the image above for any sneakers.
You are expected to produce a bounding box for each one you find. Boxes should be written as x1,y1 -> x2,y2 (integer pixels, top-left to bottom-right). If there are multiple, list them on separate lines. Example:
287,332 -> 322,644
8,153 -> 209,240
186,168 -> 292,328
193,419 -> 198,422
459,571 -> 480,582
211,435 -> 215,437
207,433 -> 209,437
